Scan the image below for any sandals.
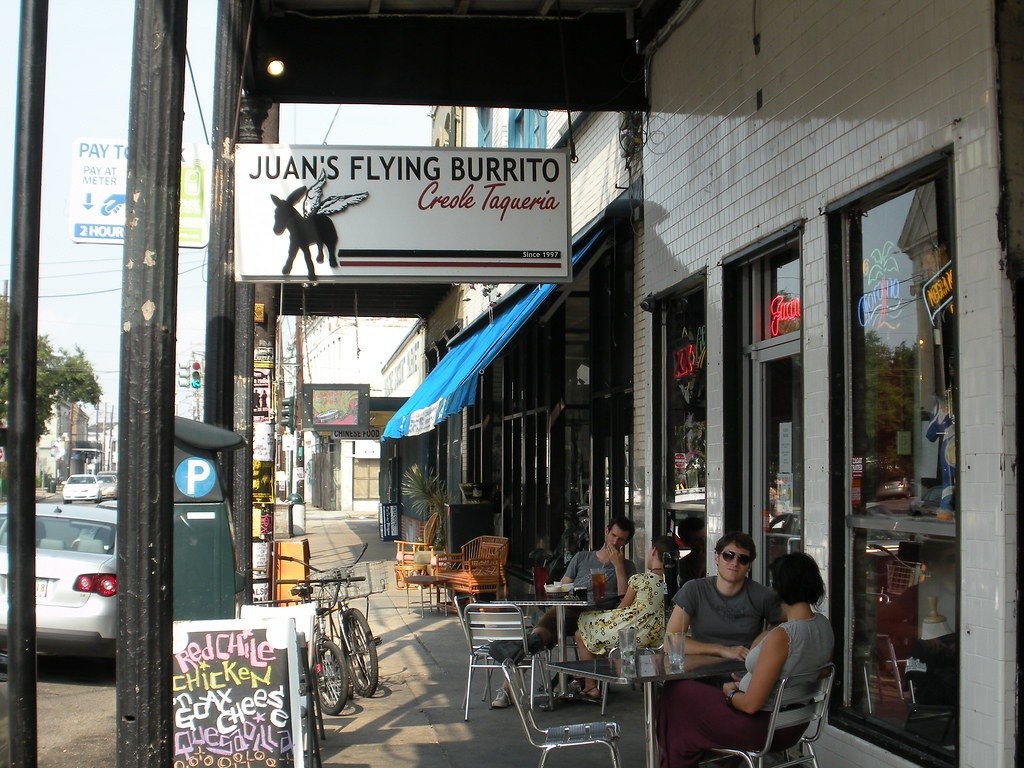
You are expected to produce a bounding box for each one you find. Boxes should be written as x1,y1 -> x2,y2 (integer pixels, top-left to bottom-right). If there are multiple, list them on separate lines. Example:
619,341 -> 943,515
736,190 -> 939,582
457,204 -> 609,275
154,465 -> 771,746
580,686 -> 603,700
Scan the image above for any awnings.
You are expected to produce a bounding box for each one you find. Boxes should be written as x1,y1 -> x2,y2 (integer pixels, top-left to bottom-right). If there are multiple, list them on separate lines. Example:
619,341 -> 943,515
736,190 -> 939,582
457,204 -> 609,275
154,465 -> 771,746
380,222 -> 607,443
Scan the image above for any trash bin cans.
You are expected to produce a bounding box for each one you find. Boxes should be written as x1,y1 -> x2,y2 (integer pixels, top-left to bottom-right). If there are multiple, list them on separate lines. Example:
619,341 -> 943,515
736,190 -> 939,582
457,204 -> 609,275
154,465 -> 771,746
287,494 -> 306,536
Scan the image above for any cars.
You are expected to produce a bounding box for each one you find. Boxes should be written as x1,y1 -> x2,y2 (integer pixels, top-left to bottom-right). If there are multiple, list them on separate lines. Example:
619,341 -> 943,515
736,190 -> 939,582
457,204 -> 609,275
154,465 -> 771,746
0,501 -> 122,670
61,474 -> 102,504
95,475 -> 118,499
95,502 -> 118,511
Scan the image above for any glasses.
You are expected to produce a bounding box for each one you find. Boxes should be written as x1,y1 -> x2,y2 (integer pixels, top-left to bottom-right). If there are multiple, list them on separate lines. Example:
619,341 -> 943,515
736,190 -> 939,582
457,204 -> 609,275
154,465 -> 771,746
720,551 -> 750,565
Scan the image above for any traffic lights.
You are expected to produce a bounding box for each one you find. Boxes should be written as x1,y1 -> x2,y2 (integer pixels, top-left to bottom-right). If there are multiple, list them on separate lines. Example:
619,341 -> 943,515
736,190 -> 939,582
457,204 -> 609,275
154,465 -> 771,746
281,398 -> 290,426
178,361 -> 190,387
191,361 -> 201,388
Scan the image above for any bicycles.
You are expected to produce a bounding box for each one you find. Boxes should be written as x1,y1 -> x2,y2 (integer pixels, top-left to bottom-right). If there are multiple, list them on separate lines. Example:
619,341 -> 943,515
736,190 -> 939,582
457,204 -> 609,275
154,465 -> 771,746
276,541 -> 382,716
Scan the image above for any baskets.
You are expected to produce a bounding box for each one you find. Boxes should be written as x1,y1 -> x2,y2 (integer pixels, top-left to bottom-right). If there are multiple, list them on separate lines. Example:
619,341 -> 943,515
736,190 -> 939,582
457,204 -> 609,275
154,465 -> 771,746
546,592 -> 569,598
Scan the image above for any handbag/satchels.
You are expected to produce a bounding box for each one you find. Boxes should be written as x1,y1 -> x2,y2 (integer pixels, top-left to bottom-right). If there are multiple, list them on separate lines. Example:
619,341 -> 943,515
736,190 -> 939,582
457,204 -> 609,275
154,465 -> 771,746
489,631 -> 544,667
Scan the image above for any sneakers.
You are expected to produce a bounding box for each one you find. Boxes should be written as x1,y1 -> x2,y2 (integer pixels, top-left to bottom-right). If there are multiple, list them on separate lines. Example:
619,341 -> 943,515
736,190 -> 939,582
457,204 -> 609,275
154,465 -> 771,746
492,689 -> 512,707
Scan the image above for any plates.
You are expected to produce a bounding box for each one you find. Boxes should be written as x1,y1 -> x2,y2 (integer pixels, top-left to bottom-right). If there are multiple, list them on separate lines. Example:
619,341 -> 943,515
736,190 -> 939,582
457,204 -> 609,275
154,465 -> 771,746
546,592 -> 569,597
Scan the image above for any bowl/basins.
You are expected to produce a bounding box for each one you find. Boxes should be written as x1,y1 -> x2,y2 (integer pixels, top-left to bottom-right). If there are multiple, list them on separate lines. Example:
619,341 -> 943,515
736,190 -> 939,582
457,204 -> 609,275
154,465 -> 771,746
544,585 -> 572,592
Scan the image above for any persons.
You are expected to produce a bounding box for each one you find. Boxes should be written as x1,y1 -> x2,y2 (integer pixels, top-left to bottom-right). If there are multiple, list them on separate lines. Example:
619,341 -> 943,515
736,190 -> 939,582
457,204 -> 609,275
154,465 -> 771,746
88,463 -> 95,474
663,531 -> 788,688
768,487 -> 776,519
655,552 -> 834,768
572,536 -> 682,702
663,655 -> 727,674
491,516 -> 637,708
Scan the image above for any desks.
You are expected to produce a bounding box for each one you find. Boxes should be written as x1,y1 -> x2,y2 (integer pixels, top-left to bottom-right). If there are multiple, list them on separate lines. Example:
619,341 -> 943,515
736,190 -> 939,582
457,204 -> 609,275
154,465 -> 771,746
479,590 -> 623,711
546,648 -> 746,768
406,577 -> 450,617
395,563 -> 425,590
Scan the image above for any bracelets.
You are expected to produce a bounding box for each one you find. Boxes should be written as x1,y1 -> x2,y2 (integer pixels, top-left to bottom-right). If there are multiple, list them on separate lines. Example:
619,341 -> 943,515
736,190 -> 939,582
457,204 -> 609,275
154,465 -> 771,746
726,689 -> 746,712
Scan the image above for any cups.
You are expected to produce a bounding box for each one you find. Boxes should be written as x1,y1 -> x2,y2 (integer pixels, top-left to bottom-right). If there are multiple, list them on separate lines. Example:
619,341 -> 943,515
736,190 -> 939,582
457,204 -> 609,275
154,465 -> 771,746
573,587 -> 587,596
666,632 -> 686,665
532,566 -> 549,595
590,568 -> 606,598
620,659 -> 638,678
617,628 -> 636,659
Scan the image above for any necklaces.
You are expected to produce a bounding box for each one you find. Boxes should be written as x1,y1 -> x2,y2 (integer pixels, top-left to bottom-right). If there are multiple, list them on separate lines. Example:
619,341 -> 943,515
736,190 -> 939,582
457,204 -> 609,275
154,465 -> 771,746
651,567 -> 663,571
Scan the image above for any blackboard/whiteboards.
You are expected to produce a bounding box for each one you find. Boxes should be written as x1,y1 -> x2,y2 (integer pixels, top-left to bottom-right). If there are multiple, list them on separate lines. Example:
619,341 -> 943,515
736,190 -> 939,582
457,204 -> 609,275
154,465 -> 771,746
171,616 -> 304,767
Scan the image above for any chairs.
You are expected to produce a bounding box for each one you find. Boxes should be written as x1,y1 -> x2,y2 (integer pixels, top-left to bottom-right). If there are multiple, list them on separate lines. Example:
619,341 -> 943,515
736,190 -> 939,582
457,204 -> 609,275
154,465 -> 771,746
36,520 -> 111,552
394,511 -> 838,768
